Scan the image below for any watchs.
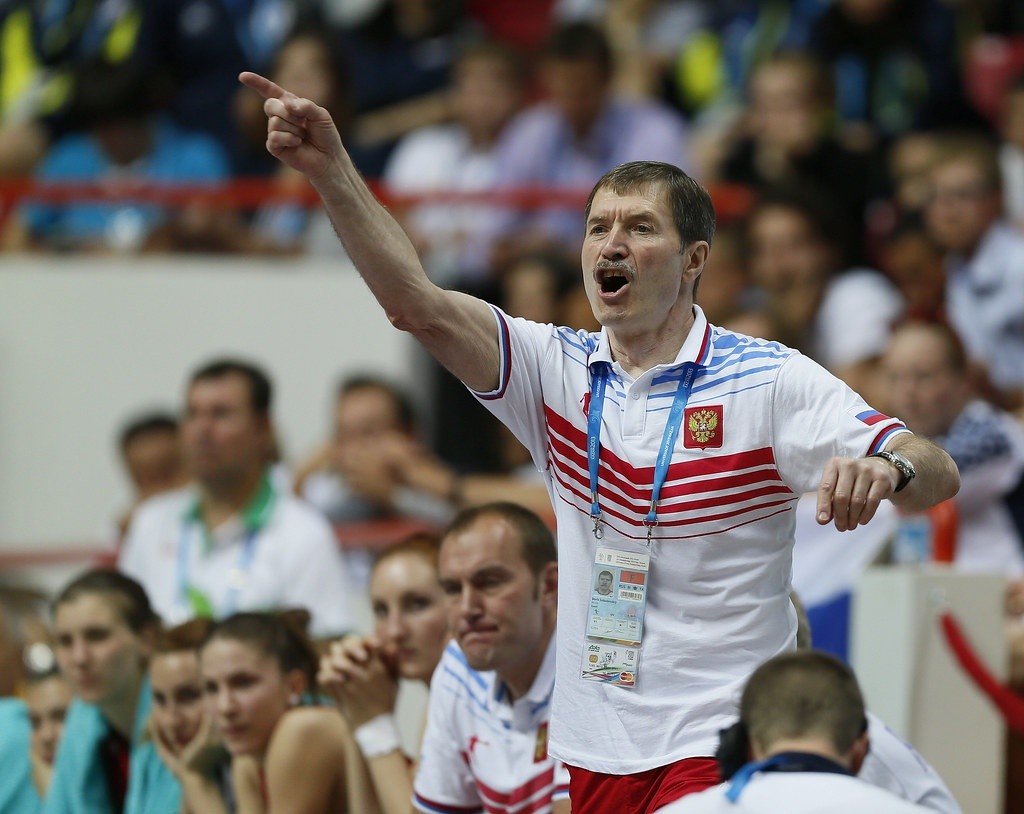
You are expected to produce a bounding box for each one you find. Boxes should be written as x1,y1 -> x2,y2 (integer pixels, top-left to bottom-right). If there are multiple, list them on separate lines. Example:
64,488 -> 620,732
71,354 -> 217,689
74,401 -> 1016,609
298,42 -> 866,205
869,450 -> 915,493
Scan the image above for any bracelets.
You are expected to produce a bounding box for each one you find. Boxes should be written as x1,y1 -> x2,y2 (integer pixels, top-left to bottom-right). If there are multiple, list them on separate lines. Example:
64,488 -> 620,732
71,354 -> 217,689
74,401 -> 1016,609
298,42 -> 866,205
450,470 -> 463,501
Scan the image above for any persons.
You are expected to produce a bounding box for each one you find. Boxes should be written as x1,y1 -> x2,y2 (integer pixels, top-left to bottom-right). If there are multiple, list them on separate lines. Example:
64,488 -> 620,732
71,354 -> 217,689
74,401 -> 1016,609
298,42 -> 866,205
0,0 -> 1024,570
238,71 -> 959,814
0,359 -> 559,814
650,652 -> 938,814
